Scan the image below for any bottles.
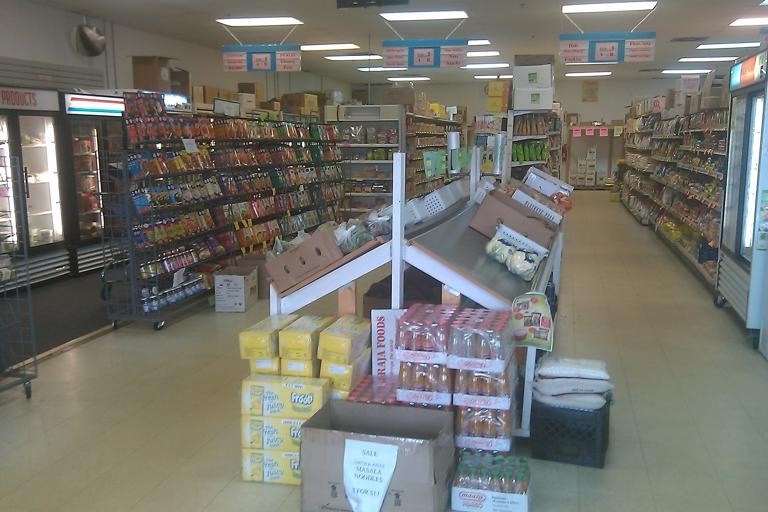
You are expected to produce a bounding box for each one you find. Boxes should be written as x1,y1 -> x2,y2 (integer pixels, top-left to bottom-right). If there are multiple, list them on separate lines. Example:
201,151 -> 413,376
393,300 -> 534,509
122,91 -> 346,316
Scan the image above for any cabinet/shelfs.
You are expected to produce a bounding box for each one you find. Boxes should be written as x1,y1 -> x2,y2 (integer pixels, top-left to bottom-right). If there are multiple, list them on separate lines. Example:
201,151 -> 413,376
619,128 -> 727,289
501,108 -> 562,188
330,120 -> 474,213
97,110 -> 350,331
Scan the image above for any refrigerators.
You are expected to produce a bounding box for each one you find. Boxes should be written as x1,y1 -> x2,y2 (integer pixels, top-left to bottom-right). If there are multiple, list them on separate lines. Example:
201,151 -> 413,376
717,47 -> 768,334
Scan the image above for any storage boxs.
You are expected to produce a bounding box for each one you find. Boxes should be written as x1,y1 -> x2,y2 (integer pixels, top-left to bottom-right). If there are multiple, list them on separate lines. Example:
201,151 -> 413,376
236,313 -> 372,487
451,483 -> 531,512
297,399 -> 453,512
428,103 -> 447,119
486,64 -> 554,113
635,69 -> 720,113
468,163 -> 575,246
191,85 -> 318,116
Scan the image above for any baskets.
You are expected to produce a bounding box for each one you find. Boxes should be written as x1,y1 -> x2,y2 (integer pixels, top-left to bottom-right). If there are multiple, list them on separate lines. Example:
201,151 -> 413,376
520,165 -> 575,200
511,187 -> 563,226
377,195 -> 429,230
421,178 -> 467,215
474,180 -> 498,206
493,221 -> 551,261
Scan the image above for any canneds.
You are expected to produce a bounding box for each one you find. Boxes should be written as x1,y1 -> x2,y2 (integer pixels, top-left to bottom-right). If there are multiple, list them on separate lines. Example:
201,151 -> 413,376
139,248 -> 206,314
